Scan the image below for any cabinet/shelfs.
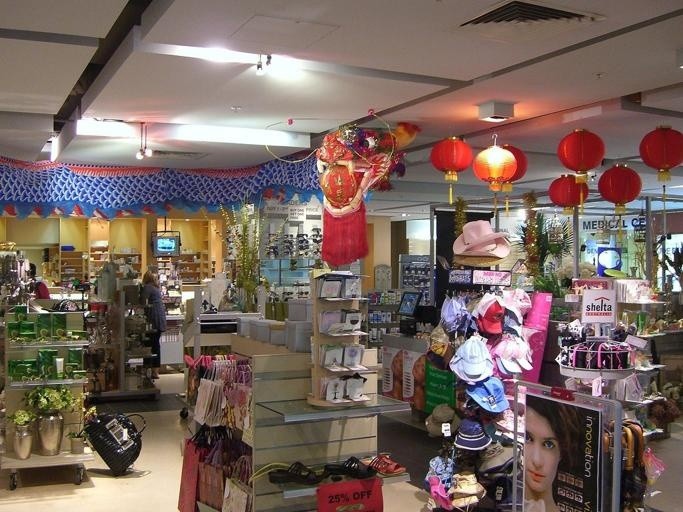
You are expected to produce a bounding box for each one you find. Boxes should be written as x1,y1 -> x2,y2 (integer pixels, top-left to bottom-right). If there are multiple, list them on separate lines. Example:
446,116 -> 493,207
306,267 -> 383,411
124,304 -> 157,358
368,304 -> 401,347
257,393 -> 411,500
617,299 -> 668,438
59,251 -> 208,283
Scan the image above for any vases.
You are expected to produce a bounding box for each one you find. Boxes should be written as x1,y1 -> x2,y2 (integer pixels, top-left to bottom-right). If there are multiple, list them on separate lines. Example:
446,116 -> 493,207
70,437 -> 84,454
36,410 -> 64,456
12,425 -> 33,459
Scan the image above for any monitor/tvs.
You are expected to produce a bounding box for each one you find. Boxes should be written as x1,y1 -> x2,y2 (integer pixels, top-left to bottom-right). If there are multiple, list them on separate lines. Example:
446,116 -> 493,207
152,236 -> 180,256
397,292 -> 422,315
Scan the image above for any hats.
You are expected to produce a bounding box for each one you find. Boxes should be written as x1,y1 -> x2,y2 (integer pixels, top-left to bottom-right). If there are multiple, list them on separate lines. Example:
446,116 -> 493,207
424,405 -> 461,437
452,220 -> 513,268
424,441 -> 513,512
441,287 -> 534,417
42,299 -> 80,312
454,418 -> 493,451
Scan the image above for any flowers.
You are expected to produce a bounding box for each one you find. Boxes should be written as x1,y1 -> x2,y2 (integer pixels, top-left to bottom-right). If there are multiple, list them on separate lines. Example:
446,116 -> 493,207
19,385 -> 75,415
64,392 -> 97,437
5,409 -> 39,426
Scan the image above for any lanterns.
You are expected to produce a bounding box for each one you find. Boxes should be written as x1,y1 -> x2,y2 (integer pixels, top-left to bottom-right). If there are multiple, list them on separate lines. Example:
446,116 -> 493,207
598,163 -> 642,241
548,173 -> 589,238
498,142 -> 529,216
558,127 -> 604,217
430,135 -> 472,207
638,125 -> 683,201
319,162 -> 364,209
472,133 -> 517,217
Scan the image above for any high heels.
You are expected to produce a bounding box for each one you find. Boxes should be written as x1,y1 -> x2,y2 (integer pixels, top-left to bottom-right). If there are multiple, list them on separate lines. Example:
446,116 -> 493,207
325,456 -> 377,478
360,454 -> 407,476
268,462 -> 324,484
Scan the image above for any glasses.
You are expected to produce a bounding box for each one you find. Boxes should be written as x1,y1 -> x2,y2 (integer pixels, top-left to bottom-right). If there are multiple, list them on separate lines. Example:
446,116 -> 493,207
265,228 -> 323,256
296,292 -> 309,295
282,292 -> 293,295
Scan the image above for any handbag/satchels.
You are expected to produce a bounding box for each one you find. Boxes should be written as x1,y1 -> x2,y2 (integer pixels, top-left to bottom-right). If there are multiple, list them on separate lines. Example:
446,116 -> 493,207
84,411 -> 141,476
178,364 -> 252,512
201,300 -> 217,313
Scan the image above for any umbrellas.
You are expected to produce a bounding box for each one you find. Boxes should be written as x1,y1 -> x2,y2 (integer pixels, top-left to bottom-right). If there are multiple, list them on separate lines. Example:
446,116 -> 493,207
602,417 -> 666,511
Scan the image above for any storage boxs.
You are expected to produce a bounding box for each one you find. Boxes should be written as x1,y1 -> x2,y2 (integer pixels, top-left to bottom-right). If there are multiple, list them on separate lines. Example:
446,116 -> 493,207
236,298 -> 312,353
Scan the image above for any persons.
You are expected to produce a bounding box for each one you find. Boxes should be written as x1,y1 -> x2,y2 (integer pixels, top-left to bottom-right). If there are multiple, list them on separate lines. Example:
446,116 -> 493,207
525,395 -> 565,511
27,263 -> 50,300
138,270 -> 168,379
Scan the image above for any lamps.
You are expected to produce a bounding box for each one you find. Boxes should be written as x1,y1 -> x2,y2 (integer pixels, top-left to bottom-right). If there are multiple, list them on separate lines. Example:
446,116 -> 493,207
136,122 -> 152,160
477,101 -> 513,123
256,53 -> 272,76
